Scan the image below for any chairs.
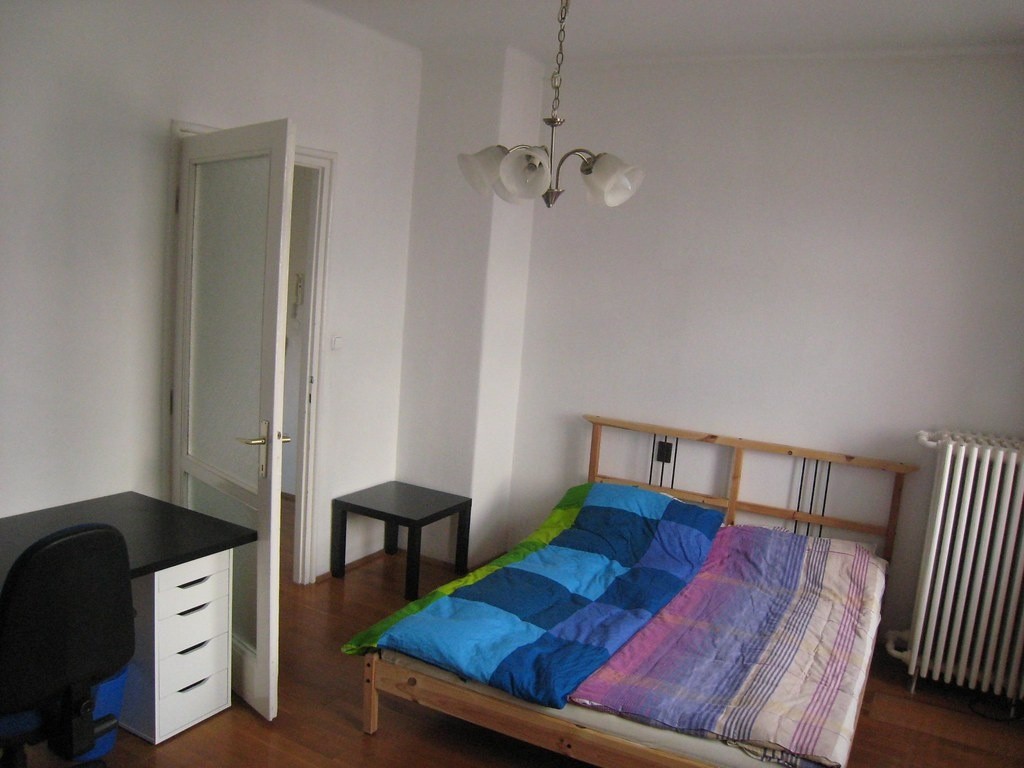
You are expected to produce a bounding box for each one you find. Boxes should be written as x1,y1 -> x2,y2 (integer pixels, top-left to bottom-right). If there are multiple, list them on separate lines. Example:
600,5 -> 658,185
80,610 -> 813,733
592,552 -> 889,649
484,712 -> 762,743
1,521 -> 137,768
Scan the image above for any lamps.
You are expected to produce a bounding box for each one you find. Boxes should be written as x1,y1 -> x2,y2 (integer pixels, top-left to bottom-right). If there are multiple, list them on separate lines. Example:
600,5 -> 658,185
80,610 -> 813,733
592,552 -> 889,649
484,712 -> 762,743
458,0 -> 652,221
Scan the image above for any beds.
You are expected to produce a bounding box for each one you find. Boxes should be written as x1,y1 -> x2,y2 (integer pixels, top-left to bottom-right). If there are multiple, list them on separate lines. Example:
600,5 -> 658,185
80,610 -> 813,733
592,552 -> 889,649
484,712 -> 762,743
342,413 -> 922,767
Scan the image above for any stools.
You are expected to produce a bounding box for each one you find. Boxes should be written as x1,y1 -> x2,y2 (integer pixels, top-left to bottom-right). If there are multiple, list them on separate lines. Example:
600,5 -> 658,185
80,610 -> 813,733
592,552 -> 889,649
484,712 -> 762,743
329,479 -> 474,601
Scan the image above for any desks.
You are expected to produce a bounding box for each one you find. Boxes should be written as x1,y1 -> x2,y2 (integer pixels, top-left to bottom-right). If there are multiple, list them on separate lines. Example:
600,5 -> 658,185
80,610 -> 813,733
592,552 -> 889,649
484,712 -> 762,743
1,489 -> 259,751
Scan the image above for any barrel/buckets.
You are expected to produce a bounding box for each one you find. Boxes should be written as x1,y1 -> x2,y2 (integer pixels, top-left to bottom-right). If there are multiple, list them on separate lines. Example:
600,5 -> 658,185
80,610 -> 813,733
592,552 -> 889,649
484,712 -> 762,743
73,664 -> 130,762
